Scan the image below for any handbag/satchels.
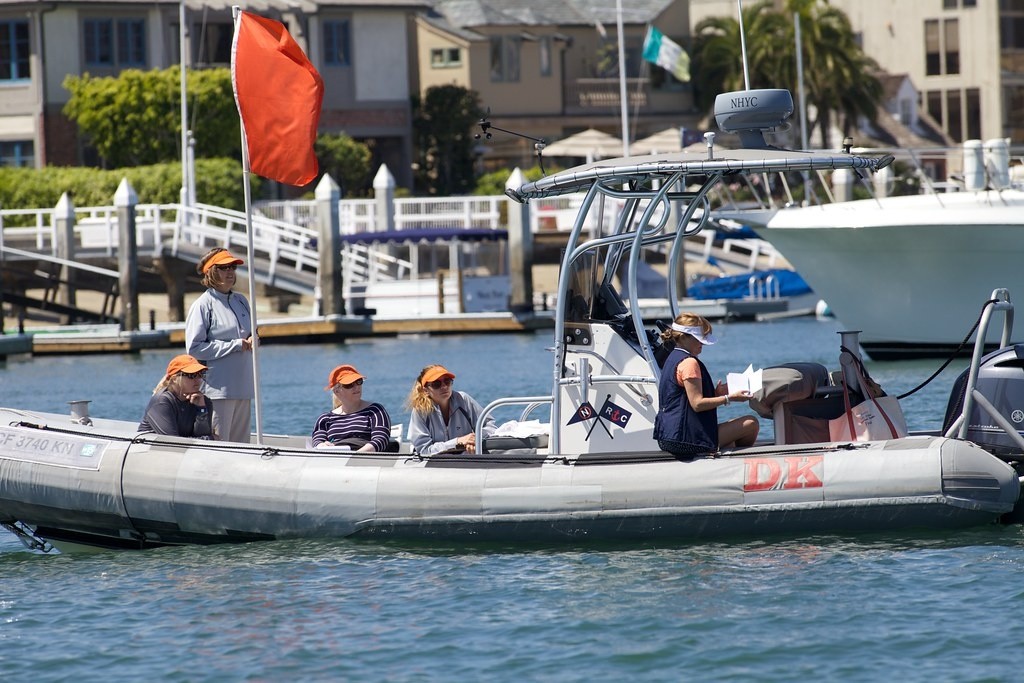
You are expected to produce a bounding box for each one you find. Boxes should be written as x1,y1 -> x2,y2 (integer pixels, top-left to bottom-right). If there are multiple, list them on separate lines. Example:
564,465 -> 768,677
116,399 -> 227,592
828,351 -> 907,441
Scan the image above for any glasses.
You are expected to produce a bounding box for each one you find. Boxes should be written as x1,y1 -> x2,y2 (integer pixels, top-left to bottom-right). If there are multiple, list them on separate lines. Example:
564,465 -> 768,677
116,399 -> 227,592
426,377 -> 453,389
217,263 -> 237,271
175,372 -> 203,379
342,378 -> 363,389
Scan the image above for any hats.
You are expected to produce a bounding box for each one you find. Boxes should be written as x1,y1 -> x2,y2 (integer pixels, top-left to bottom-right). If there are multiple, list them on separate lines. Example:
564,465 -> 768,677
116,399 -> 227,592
672,323 -> 719,346
422,366 -> 456,384
329,364 -> 368,384
202,250 -> 245,274
165,354 -> 209,380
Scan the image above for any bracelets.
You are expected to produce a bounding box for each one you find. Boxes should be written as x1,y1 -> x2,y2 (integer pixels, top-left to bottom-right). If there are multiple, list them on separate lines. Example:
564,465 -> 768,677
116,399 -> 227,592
725,395 -> 730,406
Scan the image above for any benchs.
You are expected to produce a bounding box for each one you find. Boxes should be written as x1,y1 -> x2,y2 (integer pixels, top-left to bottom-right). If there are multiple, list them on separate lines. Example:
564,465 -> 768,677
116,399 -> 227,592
474,395 -> 552,455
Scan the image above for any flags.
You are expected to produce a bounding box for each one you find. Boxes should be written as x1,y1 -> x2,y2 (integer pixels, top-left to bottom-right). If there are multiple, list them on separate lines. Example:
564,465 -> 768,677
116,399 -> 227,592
230,10 -> 324,186
641,25 -> 691,82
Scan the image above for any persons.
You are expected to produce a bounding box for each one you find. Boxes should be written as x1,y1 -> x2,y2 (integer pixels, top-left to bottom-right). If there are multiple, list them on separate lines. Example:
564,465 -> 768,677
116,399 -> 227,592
653,312 -> 760,455
405,365 -> 498,457
137,354 -> 219,441
185,247 -> 260,444
312,364 -> 391,452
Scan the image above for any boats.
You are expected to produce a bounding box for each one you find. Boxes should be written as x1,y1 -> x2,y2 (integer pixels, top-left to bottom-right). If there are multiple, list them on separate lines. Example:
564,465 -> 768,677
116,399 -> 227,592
1,1 -> 1024,550
707,138 -> 1024,364
